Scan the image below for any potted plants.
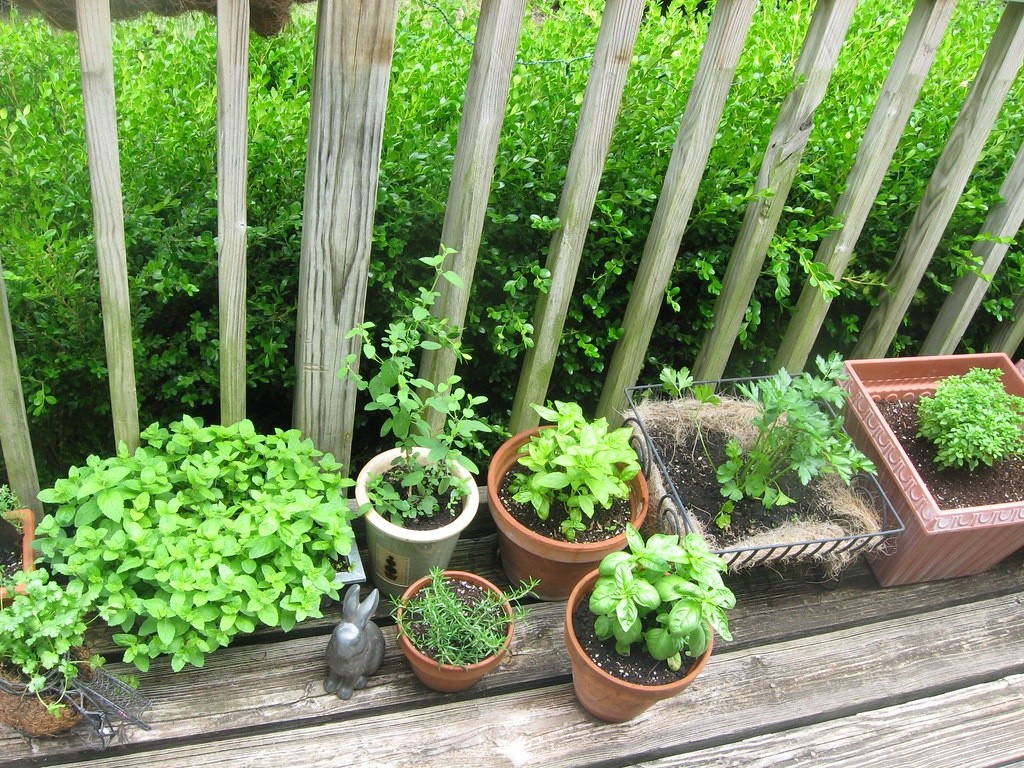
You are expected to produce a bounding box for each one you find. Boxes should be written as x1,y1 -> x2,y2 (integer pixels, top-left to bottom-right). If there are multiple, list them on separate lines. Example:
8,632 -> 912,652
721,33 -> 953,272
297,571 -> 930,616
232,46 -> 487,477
562,522 -> 739,723
337,241 -> 511,586
825,353 -> 1024,588
615,349 -> 906,591
0,484 -> 37,607
33,413 -> 368,673
389,566 -> 545,693
485,397 -> 652,600
